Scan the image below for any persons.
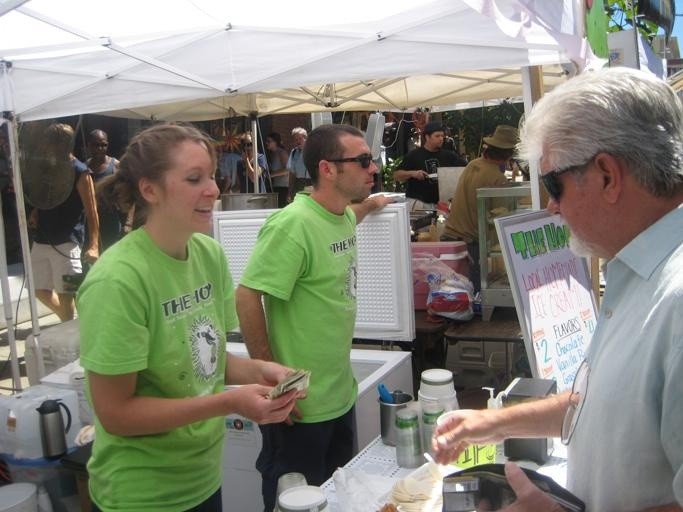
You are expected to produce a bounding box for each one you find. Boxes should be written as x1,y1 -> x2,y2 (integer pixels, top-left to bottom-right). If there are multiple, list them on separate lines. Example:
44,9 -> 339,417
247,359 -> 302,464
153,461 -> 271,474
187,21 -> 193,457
430,67 -> 682,512
75,124 -> 307,511
235,123 -> 399,512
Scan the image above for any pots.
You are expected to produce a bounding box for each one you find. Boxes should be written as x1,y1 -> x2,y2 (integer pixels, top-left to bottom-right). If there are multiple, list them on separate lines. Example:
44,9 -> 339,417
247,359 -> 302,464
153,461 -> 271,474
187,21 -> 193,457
218,193 -> 278,211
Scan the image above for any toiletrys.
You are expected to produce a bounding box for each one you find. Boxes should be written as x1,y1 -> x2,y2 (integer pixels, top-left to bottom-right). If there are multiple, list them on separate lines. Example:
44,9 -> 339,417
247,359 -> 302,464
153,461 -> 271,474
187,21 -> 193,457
481,384 -> 497,406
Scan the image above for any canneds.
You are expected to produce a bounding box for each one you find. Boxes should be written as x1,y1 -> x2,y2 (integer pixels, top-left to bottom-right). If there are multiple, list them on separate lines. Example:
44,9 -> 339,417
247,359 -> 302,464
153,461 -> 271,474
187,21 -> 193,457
422,408 -> 445,453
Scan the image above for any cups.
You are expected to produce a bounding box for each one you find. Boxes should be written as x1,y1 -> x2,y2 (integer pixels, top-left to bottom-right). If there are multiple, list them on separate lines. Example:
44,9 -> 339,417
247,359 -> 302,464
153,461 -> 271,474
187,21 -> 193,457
275,473 -> 306,512
278,486 -> 328,512
377,388 -> 413,445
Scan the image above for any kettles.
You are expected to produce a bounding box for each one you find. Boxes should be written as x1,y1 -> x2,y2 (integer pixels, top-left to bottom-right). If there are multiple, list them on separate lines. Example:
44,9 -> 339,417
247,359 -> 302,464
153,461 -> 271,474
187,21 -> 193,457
422,405 -> 446,463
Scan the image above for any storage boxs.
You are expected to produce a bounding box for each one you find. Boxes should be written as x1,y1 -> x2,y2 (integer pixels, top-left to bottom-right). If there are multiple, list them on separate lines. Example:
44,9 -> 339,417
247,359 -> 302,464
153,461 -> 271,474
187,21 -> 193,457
25,316 -> 79,385
410,242 -> 468,312
1,384 -> 82,462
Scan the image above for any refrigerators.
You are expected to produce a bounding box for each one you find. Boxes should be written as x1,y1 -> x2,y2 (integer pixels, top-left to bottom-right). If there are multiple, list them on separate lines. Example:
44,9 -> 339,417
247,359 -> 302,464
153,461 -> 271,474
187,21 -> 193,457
38,202 -> 415,510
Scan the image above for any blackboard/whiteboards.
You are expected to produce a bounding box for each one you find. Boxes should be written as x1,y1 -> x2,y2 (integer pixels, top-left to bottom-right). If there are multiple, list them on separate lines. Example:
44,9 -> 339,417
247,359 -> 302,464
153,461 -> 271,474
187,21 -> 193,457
493,209 -> 600,399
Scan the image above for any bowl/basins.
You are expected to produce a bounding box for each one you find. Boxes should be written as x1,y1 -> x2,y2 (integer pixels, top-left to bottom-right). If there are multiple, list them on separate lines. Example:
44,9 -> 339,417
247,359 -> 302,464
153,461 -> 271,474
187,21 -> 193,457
417,367 -> 458,412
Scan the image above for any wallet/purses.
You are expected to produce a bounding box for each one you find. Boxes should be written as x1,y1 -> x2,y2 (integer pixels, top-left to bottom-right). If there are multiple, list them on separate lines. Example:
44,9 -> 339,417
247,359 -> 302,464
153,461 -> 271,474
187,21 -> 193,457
441,461 -> 587,512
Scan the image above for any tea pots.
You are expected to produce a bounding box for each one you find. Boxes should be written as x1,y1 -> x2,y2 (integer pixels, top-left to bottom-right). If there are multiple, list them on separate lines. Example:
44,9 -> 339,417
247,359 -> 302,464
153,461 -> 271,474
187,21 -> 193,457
35,398 -> 72,460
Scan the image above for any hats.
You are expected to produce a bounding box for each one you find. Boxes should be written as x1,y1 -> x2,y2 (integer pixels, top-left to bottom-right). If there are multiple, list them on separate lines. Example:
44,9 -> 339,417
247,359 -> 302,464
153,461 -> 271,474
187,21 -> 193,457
425,120 -> 442,132
482,123 -> 522,152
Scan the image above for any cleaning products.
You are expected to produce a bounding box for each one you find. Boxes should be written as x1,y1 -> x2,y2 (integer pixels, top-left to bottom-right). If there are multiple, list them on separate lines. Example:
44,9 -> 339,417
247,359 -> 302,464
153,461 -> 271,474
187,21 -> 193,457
417,405 -> 446,455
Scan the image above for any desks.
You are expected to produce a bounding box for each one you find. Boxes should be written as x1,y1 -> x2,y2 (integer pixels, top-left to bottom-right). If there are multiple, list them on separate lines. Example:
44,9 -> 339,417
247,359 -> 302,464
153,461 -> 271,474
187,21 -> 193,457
445,313 -> 526,395
413,309 -> 452,401
271,434 -> 571,511
59,439 -> 93,511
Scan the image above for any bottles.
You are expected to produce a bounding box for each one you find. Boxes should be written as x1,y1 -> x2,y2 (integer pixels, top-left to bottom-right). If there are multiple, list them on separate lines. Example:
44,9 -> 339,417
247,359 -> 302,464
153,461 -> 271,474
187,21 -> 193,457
36,485 -> 51,510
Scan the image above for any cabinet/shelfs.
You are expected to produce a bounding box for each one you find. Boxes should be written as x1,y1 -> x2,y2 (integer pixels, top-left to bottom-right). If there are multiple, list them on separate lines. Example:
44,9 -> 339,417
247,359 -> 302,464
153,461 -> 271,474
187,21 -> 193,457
475,186 -> 534,322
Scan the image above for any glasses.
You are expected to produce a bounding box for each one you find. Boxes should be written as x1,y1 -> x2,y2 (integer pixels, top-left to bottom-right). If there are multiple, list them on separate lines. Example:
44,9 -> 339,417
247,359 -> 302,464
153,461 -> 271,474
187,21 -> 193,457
326,155 -> 373,167
242,142 -> 252,147
559,355 -> 592,448
540,149 -> 613,200
